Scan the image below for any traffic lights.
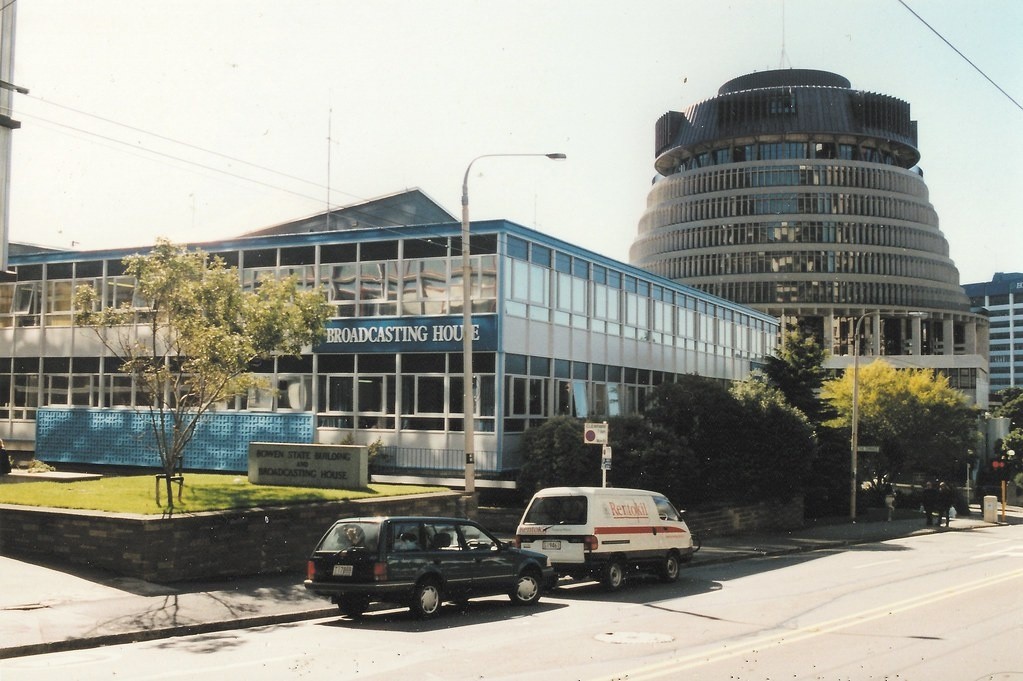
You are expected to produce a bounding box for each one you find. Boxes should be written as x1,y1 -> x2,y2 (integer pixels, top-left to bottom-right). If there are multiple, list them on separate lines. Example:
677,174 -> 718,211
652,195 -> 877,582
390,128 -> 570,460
990,461 -> 1005,470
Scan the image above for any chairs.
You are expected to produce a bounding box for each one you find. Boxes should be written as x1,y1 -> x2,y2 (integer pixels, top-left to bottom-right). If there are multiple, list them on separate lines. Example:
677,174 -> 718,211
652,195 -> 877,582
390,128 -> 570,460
434,533 -> 451,551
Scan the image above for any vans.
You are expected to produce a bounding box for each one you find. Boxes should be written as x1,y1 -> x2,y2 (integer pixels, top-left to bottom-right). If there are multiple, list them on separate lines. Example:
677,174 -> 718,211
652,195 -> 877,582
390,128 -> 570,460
513,487 -> 703,592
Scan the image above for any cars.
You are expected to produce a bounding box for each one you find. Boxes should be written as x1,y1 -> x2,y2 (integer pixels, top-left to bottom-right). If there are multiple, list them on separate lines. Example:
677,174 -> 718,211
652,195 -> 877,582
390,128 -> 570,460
302,515 -> 557,617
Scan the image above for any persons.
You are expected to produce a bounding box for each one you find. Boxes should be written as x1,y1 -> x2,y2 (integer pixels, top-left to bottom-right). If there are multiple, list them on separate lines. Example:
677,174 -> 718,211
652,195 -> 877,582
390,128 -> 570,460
935,482 -> 955,528
919,480 -> 938,527
393,532 -> 417,551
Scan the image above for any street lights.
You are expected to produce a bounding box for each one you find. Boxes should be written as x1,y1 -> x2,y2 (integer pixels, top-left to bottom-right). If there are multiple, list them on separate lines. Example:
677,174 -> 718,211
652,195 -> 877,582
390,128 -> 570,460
849,310 -> 929,519
460,150 -> 567,501
